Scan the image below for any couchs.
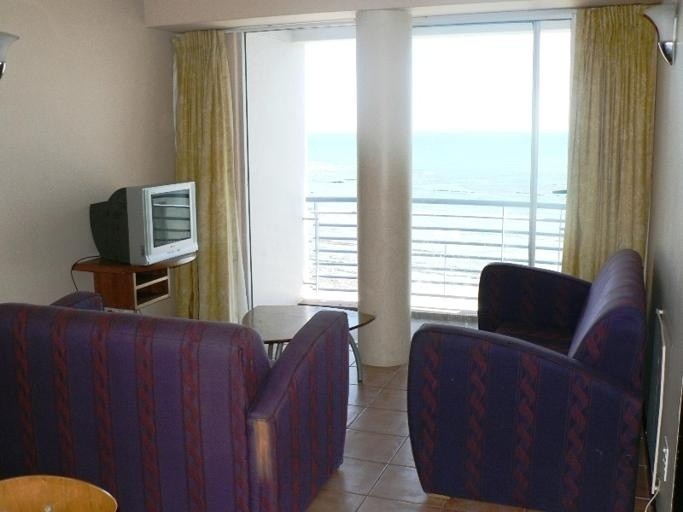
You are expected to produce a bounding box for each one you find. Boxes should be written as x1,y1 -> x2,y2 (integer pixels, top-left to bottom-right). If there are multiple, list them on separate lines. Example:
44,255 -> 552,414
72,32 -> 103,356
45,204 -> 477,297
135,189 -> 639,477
406,248 -> 652,512
0,302 -> 352,511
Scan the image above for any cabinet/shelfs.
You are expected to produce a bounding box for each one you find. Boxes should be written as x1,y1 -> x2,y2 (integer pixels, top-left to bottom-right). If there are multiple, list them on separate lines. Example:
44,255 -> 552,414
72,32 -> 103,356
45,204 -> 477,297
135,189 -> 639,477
77,259 -> 201,310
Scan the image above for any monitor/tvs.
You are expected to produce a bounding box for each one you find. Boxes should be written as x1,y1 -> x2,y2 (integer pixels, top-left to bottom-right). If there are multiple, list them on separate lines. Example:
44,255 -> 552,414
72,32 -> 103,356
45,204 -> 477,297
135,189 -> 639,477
89,181 -> 199,265
